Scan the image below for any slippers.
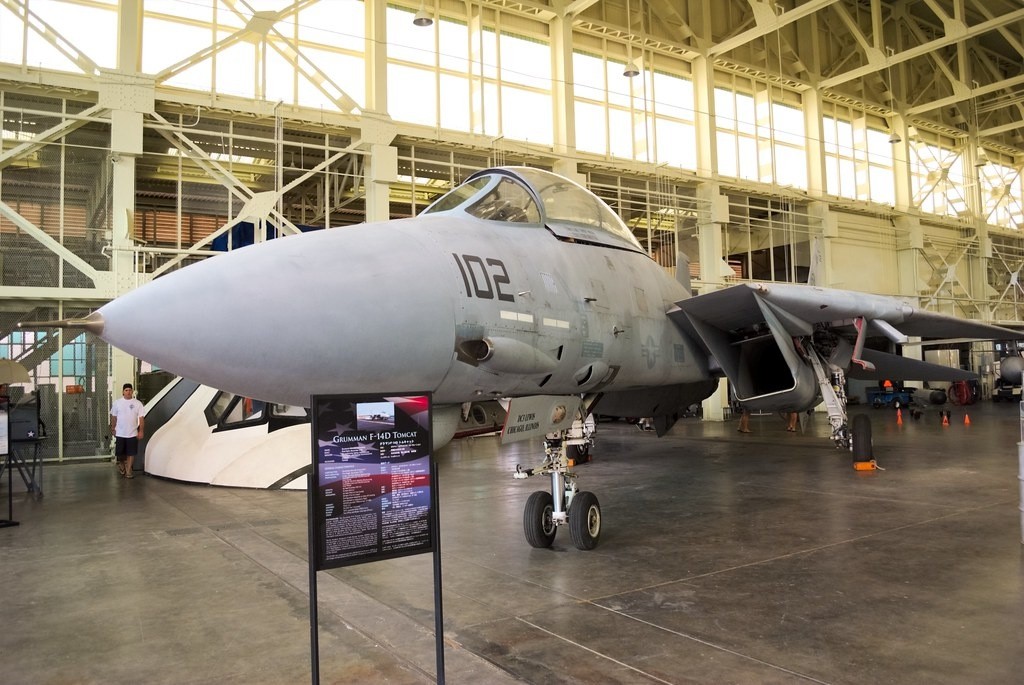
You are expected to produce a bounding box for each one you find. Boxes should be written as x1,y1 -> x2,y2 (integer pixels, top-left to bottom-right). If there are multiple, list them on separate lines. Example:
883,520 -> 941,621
126,474 -> 134,479
119,468 -> 126,475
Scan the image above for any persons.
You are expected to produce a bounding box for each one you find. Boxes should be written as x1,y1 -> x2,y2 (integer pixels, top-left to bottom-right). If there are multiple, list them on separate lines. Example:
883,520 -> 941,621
635,417 -> 657,433
109,383 -> 145,479
737,410 -> 752,435
787,412 -> 798,432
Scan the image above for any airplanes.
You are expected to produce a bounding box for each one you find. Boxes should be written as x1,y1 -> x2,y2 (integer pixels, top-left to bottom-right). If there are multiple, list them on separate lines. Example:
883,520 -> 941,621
16,168 -> 1024,552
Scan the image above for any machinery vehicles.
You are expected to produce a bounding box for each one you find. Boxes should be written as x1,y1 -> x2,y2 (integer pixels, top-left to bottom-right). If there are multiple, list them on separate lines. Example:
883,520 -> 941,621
870,380 -> 911,409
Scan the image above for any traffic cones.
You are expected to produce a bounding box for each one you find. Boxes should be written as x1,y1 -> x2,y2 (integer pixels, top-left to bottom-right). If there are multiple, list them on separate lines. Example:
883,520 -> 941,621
964,414 -> 970,424
896,408 -> 903,425
941,415 -> 949,426
